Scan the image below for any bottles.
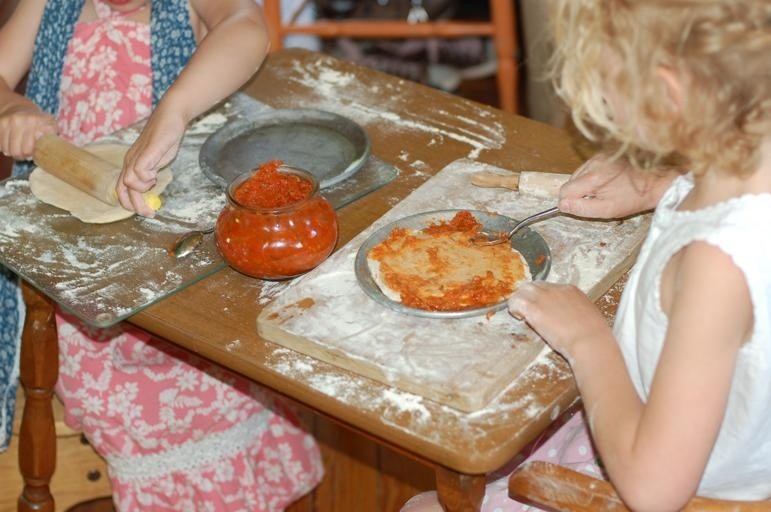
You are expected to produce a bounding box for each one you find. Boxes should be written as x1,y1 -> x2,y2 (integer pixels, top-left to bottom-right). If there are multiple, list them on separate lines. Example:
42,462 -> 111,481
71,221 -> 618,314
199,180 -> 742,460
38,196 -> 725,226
215,165 -> 339,280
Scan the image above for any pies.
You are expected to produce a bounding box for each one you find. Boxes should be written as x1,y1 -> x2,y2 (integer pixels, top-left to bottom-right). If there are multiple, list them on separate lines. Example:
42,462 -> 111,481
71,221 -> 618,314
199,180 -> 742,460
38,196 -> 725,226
28,144 -> 173,224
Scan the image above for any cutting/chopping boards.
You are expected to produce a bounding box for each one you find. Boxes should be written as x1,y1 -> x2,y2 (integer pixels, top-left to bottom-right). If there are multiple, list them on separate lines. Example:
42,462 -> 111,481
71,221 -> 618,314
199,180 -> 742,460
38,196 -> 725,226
0,93 -> 400,331
255,156 -> 651,415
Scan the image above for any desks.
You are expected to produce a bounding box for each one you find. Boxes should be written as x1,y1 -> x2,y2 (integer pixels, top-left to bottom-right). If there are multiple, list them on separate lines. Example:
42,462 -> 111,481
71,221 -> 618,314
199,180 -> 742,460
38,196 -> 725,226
0,47 -> 675,512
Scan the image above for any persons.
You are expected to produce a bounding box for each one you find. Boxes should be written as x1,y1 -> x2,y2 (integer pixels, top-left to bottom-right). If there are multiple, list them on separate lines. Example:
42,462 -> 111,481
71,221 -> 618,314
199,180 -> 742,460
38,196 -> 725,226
400,1 -> 771,512
0,0 -> 326,512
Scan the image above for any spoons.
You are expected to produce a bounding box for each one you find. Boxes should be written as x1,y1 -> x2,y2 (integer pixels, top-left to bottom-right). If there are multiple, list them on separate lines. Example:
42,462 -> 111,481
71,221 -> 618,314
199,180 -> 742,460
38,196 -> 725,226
169,228 -> 216,259
474,193 -> 595,246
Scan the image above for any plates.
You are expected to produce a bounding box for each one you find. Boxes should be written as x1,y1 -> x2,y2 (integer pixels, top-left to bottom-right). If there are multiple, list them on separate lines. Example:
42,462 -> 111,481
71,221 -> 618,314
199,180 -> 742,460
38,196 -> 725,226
354,208 -> 553,317
199,108 -> 371,192
